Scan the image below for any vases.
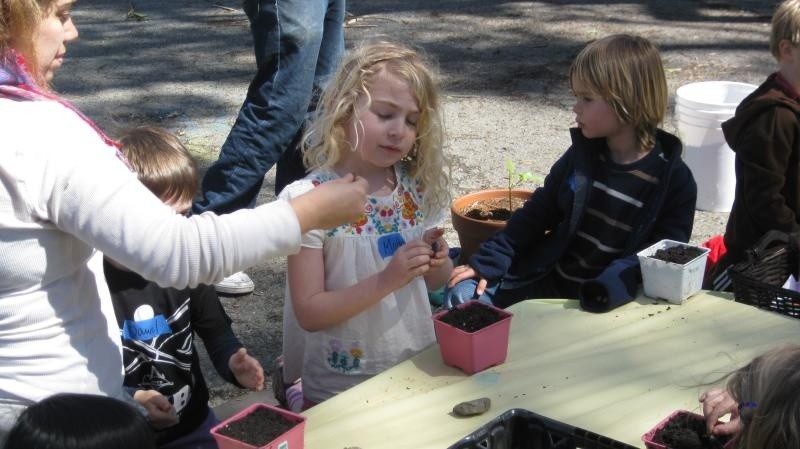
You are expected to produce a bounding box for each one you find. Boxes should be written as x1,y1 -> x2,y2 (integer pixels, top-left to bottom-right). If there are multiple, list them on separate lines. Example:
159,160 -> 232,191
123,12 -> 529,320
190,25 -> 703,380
209,402 -> 307,449
640,409 -> 740,449
434,300 -> 514,374
637,238 -> 712,304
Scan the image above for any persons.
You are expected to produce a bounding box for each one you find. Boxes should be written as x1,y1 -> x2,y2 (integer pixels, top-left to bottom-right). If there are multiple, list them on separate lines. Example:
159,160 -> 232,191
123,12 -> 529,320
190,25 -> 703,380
704,0 -> 800,293
444,33 -> 697,314
277,42 -> 456,424
0,0 -> 371,449
698,342 -> 800,449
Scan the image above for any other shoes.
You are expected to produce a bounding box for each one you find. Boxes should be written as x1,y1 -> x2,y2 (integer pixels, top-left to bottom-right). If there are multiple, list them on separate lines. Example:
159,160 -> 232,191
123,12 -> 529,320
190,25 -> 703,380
270,355 -> 297,406
214,272 -> 254,294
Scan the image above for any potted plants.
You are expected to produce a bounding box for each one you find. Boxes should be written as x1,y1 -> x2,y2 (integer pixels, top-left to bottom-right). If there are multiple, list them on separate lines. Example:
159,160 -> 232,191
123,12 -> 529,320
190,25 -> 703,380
451,160 -> 538,266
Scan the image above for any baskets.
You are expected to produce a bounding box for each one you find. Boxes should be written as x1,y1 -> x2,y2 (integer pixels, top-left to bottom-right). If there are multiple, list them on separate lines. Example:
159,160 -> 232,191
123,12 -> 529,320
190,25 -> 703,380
726,232 -> 800,319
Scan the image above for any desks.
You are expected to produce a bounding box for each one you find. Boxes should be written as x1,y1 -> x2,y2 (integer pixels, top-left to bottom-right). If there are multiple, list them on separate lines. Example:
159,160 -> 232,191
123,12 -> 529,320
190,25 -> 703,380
298,286 -> 800,449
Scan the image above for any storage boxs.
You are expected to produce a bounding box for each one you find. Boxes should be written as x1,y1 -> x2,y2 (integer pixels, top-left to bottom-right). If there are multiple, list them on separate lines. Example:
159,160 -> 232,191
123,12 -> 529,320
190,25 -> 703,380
731,227 -> 800,319
447,408 -> 640,449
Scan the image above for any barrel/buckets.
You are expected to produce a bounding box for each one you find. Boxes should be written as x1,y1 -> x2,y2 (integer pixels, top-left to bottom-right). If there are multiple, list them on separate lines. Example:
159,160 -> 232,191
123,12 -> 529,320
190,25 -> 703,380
673,81 -> 759,212
